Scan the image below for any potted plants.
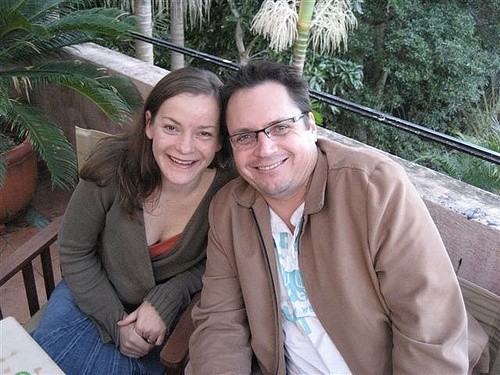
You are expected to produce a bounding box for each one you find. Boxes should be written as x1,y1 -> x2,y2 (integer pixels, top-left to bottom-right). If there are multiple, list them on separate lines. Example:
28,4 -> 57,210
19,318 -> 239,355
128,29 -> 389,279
0,1 -> 145,222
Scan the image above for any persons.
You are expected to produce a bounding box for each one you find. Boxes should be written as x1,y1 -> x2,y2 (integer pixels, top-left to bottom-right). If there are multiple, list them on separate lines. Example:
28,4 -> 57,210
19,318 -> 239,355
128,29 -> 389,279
32,68 -> 233,375
184,60 -> 490,375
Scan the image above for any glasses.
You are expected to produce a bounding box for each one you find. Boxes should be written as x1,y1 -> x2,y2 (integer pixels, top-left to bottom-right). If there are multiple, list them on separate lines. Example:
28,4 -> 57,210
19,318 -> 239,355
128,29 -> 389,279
227,113 -> 306,150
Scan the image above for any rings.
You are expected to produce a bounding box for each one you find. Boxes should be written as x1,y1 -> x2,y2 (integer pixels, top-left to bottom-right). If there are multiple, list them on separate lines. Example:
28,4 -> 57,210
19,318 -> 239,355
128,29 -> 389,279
146,338 -> 155,343
